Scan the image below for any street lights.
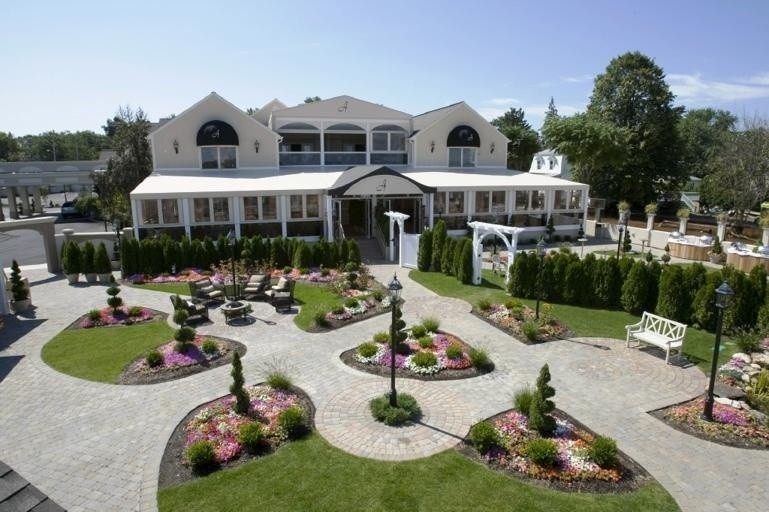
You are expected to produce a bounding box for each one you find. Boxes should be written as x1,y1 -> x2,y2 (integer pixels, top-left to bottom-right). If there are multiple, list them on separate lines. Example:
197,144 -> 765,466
226,227 -> 237,301
699,279 -> 735,421
532,237 -> 547,319
617,224 -> 624,260
387,270 -> 403,407
437,203 -> 443,219
114,217 -> 121,253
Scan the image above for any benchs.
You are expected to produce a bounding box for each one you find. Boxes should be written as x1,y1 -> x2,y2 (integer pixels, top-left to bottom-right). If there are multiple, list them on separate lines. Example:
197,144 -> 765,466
624,311 -> 688,364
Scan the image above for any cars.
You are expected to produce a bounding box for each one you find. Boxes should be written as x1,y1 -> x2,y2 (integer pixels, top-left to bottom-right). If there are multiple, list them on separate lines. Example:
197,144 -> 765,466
61,201 -> 79,219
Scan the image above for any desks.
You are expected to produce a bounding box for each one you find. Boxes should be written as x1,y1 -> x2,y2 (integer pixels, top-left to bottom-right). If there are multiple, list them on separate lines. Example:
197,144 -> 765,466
220,300 -> 248,322
274,291 -> 291,312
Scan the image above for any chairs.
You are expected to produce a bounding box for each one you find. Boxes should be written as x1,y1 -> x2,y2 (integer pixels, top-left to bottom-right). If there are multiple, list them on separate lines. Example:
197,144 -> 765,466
188,278 -> 226,306
491,254 -> 507,278
264,276 -> 295,306
244,274 -> 271,301
170,295 -> 214,328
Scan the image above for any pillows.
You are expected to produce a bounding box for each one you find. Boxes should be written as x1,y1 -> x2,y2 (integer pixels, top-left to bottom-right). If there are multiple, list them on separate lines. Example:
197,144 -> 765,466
246,282 -> 261,288
187,301 -> 195,308
271,286 -> 283,290
201,285 -> 214,294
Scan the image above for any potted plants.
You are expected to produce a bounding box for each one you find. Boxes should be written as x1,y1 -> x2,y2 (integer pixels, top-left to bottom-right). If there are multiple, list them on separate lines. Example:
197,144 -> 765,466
61,240 -> 112,285
709,235 -> 722,264
9,260 -> 31,313
